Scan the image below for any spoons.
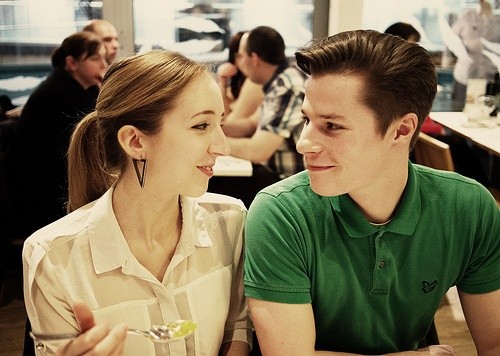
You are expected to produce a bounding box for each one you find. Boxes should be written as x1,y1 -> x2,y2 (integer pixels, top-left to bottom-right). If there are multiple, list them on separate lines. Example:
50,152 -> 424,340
29,320 -> 198,342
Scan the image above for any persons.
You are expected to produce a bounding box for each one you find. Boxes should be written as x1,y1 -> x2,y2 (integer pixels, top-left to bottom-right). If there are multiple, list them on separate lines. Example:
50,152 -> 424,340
242,31 -> 500,356
23,49 -> 258,356
0,0 -> 500,261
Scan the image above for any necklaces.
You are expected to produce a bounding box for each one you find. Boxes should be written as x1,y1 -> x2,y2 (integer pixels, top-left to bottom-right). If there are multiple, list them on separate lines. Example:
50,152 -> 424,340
370,217 -> 394,226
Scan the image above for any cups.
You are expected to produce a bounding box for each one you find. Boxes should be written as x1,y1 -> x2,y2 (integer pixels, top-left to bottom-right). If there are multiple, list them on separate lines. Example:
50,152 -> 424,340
478,95 -> 496,116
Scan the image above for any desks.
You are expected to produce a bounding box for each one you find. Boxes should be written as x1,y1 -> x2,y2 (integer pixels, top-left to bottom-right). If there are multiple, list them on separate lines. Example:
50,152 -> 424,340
429,112 -> 500,189
